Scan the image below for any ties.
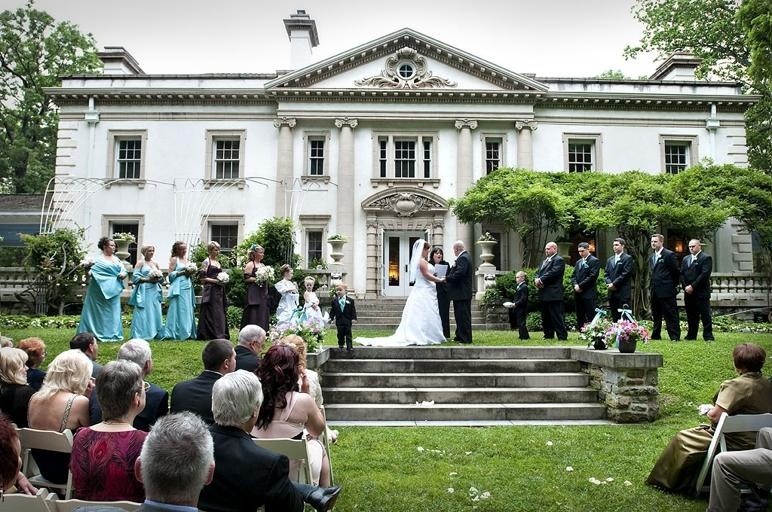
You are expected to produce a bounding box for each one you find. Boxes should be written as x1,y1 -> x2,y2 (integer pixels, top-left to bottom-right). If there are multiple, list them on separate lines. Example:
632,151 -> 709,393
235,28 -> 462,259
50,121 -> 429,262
338,297 -> 345,312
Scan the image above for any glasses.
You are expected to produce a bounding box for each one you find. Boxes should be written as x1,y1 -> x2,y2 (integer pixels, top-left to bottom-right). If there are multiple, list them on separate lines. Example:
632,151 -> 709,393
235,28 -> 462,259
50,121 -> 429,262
138,382 -> 150,392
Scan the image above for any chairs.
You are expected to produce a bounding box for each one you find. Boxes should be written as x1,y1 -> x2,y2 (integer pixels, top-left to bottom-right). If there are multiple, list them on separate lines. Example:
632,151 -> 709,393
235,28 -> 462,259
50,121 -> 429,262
9,422 -> 75,500
0,488 -> 49,512
252,436 -> 313,512
44,492 -> 143,512
696,411 -> 772,511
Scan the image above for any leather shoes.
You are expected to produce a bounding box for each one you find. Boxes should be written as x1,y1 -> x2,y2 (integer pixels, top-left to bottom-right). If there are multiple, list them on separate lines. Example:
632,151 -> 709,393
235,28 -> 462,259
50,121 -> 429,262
307,486 -> 342,512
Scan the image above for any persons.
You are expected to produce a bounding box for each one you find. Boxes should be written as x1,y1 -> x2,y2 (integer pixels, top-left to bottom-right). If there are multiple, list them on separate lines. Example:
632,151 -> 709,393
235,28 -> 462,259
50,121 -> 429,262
170,324 -> 339,487
509,272 -> 530,341
605,237 -> 634,322
76,237 -> 128,341
647,343 -> 771,493
135,410 -> 215,512
647,234 -> 681,341
705,427 -> 772,512
329,284 -> 357,350
197,369 -> 342,512
197,242 -> 230,339
428,248 -> 450,339
446,241 -> 473,343
572,242 -> 600,331
0,332 -> 168,505
275,264 -> 298,328
303,276 -> 323,326
240,245 -> 271,334
165,242 -> 195,339
400,239 -> 448,346
533,241 -> 569,340
680,239 -> 716,340
127,246 -> 166,341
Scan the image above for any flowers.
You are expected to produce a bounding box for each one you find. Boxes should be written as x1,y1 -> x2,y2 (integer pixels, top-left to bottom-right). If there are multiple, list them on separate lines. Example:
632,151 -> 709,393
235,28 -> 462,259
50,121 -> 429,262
147,270 -> 163,278
112,231 -> 137,243
267,320 -> 303,346
217,272 -> 230,283
578,317 -> 615,348
304,317 -> 327,339
601,319 -> 652,348
185,262 -> 197,272
478,232 -> 497,241
254,266 -> 274,287
327,233 -> 347,240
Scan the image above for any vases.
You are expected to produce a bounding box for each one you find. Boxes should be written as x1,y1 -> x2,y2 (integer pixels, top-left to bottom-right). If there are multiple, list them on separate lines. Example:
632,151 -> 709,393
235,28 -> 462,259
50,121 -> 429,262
617,339 -> 637,353
113,239 -> 131,259
326,239 -> 349,261
475,241 -> 498,261
594,336 -> 608,349
